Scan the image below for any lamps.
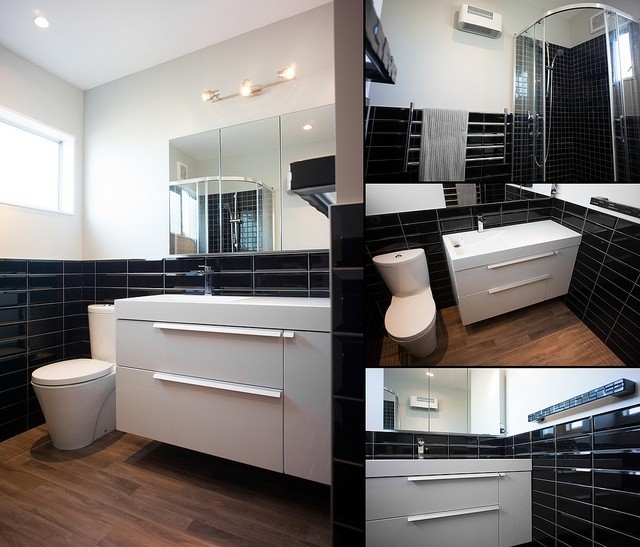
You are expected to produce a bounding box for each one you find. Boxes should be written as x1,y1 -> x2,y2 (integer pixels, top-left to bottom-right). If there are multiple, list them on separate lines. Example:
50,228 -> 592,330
241,78 -> 253,97
277,67 -> 295,81
202,90 -> 221,101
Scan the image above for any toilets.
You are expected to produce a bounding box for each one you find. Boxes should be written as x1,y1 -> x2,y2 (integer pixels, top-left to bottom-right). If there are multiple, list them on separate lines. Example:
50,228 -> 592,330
30,303 -> 115,450
371,248 -> 437,359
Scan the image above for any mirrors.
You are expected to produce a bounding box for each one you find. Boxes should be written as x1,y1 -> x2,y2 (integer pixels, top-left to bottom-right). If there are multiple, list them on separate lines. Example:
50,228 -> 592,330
382,367 -> 470,434
169,102 -> 339,255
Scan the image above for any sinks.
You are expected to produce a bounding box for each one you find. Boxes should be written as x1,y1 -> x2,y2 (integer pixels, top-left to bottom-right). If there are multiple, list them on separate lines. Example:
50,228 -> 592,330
129,294 -> 244,304
460,224 -> 565,251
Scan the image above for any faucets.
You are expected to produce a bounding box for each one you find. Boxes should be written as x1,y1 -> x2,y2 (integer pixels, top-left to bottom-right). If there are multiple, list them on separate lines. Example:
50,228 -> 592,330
198,265 -> 214,296
477,213 -> 486,233
416,437 -> 425,459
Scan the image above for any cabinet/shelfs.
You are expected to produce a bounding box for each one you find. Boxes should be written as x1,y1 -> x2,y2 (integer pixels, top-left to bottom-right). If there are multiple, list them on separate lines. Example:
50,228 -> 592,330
113,294 -> 332,487
366,459 -> 537,546
442,233 -> 581,326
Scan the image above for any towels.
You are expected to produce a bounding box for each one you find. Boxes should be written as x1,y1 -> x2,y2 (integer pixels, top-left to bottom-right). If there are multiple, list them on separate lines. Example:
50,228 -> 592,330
421,108 -> 470,181
456,184 -> 478,207
